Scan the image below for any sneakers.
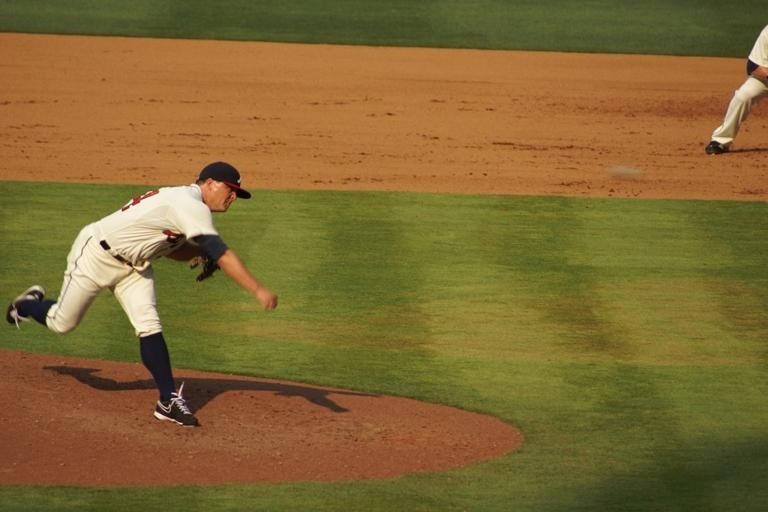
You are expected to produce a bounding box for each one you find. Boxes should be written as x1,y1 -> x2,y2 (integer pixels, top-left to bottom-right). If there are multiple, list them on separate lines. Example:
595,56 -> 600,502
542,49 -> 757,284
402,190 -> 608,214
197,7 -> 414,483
153,399 -> 198,428
7,284 -> 44,323
705,140 -> 728,155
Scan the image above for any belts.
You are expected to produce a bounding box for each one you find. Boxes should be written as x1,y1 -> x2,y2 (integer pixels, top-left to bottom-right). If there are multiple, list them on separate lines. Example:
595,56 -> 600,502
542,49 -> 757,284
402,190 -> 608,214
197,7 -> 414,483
100,239 -> 131,269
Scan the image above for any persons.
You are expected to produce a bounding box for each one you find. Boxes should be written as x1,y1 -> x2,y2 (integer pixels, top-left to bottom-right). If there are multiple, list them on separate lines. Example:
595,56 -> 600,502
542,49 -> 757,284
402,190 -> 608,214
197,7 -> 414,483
6,161 -> 277,430
704,23 -> 767,155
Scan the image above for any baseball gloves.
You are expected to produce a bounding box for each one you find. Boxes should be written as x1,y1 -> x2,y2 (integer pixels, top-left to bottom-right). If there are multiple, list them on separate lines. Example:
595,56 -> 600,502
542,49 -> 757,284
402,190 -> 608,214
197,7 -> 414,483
189,250 -> 222,282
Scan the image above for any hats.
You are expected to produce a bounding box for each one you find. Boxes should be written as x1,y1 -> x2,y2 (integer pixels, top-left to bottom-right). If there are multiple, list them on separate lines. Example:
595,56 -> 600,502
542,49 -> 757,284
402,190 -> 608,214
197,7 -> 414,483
199,160 -> 251,200
